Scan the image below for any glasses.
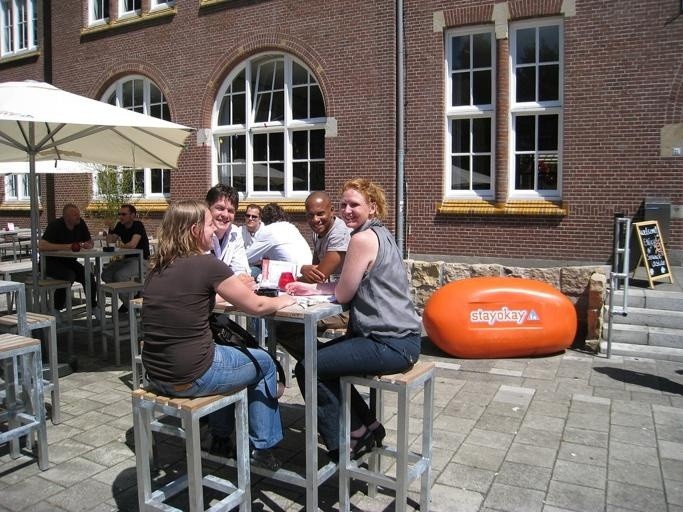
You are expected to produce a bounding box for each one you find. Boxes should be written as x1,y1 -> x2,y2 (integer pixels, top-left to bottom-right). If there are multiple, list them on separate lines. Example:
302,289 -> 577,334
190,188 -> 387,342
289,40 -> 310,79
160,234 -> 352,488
118,213 -> 130,215
245,214 -> 258,218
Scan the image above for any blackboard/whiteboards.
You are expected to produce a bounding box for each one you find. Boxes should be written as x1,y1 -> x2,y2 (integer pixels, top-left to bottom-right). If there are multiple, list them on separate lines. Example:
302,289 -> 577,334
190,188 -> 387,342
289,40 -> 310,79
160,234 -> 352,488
634,220 -> 671,281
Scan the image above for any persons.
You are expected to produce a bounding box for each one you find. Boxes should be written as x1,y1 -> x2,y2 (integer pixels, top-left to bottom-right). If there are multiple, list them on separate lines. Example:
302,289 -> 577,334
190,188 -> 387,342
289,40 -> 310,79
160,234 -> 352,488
275,192 -> 354,363
140,197 -> 286,471
37,204 -> 97,311
101,203 -> 151,313
203,184 -> 257,304
295,176 -> 423,464
237,204 -> 265,249
245,201 -> 313,343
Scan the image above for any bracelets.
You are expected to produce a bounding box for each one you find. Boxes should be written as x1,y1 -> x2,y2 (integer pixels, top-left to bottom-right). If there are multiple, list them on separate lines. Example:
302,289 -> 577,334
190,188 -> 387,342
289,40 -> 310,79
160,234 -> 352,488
81,241 -> 85,247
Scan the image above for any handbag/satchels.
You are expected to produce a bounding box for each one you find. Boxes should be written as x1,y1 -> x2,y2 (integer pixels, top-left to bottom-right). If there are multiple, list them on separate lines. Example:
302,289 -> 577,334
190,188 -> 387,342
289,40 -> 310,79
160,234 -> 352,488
208,313 -> 258,347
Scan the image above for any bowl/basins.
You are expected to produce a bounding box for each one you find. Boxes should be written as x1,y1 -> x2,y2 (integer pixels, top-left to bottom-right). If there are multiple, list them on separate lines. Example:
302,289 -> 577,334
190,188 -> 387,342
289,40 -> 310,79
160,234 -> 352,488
102,247 -> 114,252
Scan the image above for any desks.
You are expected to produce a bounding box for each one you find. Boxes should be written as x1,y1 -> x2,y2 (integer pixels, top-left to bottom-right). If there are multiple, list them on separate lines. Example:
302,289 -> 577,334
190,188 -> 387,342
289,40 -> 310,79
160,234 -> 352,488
129,292 -> 384,512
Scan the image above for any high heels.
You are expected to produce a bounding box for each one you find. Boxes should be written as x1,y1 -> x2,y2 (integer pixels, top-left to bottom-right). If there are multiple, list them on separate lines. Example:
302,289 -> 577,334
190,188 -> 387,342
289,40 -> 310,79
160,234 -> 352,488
329,428 -> 372,461
372,424 -> 386,448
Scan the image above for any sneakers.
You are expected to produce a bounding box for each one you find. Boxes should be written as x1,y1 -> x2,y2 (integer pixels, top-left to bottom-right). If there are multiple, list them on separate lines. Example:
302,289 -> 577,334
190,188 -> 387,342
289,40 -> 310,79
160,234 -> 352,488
249,445 -> 282,471
207,437 -> 236,457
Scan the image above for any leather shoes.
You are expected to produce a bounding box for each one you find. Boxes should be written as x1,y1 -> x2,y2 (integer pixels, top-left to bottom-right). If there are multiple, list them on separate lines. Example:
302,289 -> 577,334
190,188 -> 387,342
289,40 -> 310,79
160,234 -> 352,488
118,304 -> 128,312
92,296 -> 97,307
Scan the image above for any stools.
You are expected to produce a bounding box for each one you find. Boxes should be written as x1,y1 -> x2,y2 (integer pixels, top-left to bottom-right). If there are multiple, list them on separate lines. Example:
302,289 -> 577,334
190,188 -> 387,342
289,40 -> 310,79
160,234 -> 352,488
131,385 -> 252,512
0,331 -> 49,472
338,361 -> 435,511
0,226 -> 350,449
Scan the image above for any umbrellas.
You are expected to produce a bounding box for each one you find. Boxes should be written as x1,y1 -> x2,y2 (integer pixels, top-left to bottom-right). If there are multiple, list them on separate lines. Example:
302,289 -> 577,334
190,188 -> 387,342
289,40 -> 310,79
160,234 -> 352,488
0,78 -> 198,317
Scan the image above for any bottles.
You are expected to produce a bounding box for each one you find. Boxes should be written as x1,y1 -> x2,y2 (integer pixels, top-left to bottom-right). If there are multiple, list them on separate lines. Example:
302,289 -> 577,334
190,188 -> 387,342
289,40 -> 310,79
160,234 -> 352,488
278,272 -> 294,291
71,242 -> 79,251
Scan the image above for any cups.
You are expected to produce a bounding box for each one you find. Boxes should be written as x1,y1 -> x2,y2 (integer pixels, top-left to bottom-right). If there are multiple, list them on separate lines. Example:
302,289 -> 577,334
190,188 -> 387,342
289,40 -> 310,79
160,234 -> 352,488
115,239 -> 120,247
